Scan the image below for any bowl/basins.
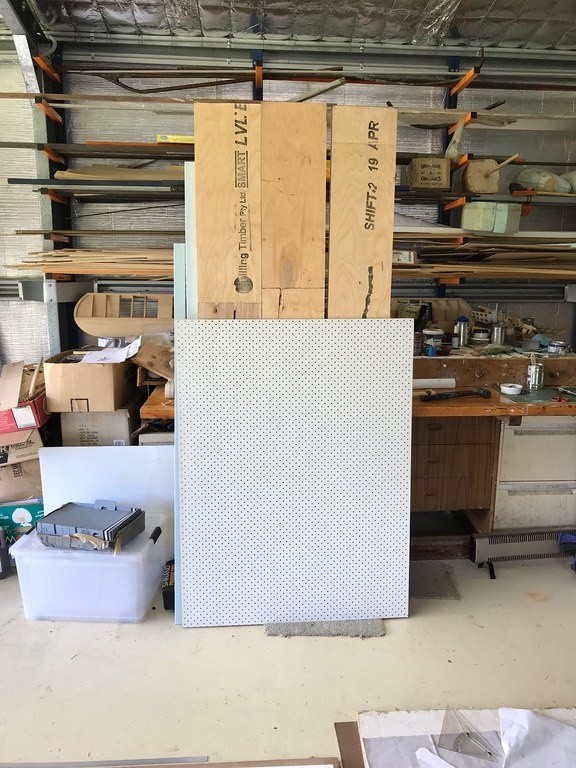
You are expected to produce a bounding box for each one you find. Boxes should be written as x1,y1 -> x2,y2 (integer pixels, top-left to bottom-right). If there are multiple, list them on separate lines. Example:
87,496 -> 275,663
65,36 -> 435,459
473,329 -> 489,339
500,383 -> 523,394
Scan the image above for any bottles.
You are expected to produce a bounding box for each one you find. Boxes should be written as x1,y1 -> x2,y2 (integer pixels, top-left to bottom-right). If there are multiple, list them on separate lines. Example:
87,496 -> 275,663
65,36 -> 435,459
432,320 -> 439,328
452,325 -> 458,349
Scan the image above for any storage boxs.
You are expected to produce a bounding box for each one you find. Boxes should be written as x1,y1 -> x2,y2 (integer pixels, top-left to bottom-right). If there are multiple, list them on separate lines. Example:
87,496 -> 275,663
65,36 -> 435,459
0,428 -> 43,467
9,512 -> 169,624
0,457 -> 43,503
0,385 -> 54,434
61,399 -> 140,448
401,157 -> 449,189
43,345 -> 128,412
461,203 -> 521,234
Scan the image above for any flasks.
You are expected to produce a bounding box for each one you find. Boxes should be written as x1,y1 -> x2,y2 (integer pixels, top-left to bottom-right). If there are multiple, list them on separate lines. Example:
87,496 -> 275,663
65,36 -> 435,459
455,321 -> 469,348
491,326 -> 505,344
526,364 -> 545,390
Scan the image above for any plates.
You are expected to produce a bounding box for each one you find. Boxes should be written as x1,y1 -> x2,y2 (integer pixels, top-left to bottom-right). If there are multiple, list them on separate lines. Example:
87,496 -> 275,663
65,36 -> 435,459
471,337 -> 490,342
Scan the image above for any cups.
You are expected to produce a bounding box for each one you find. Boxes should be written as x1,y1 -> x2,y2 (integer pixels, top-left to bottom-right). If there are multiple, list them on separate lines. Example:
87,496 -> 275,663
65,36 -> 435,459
414,332 -> 422,356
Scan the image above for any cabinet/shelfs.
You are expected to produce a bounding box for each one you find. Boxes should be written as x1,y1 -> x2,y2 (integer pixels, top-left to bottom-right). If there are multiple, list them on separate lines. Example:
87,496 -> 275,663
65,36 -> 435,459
0,93 -> 576,387
140,386 -> 576,534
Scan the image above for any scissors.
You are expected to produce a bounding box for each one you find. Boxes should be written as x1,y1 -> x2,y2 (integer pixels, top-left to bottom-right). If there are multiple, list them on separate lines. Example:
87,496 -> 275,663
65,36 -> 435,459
551,390 -> 569,403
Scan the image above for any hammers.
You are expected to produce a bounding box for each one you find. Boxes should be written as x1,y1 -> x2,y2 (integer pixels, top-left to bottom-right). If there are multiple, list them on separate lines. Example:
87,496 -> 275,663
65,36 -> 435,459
419,387 -> 492,401
